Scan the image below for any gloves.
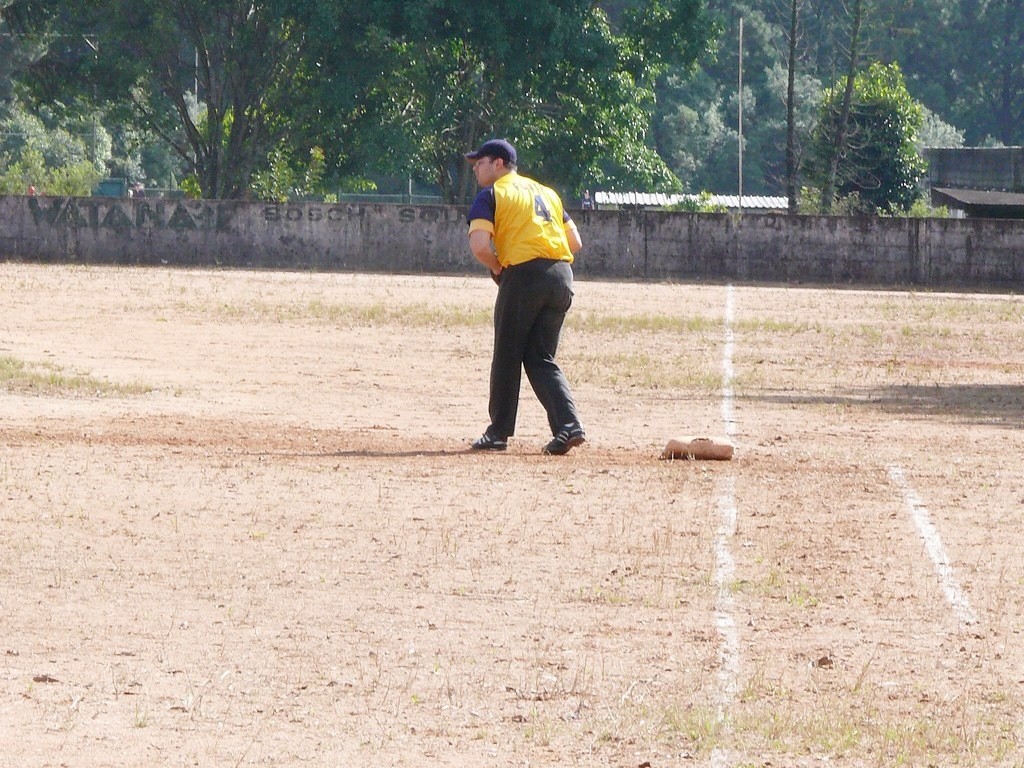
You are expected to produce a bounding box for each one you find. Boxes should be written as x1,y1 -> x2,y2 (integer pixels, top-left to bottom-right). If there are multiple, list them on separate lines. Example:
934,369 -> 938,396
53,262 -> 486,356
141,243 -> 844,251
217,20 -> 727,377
490,266 -> 503,284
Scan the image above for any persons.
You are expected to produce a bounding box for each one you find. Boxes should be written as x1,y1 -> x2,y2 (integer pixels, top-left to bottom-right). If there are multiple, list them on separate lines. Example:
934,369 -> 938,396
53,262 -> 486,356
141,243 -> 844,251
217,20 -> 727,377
465,139 -> 585,455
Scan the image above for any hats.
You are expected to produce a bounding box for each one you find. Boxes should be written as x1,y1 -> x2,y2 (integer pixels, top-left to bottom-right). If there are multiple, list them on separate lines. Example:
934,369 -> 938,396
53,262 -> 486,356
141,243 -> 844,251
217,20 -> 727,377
465,139 -> 518,163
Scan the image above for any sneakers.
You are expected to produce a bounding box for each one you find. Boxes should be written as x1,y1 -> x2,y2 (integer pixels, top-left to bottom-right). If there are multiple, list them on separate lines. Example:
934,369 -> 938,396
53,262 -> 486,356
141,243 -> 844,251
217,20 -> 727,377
541,421 -> 585,455
472,432 -> 508,450
